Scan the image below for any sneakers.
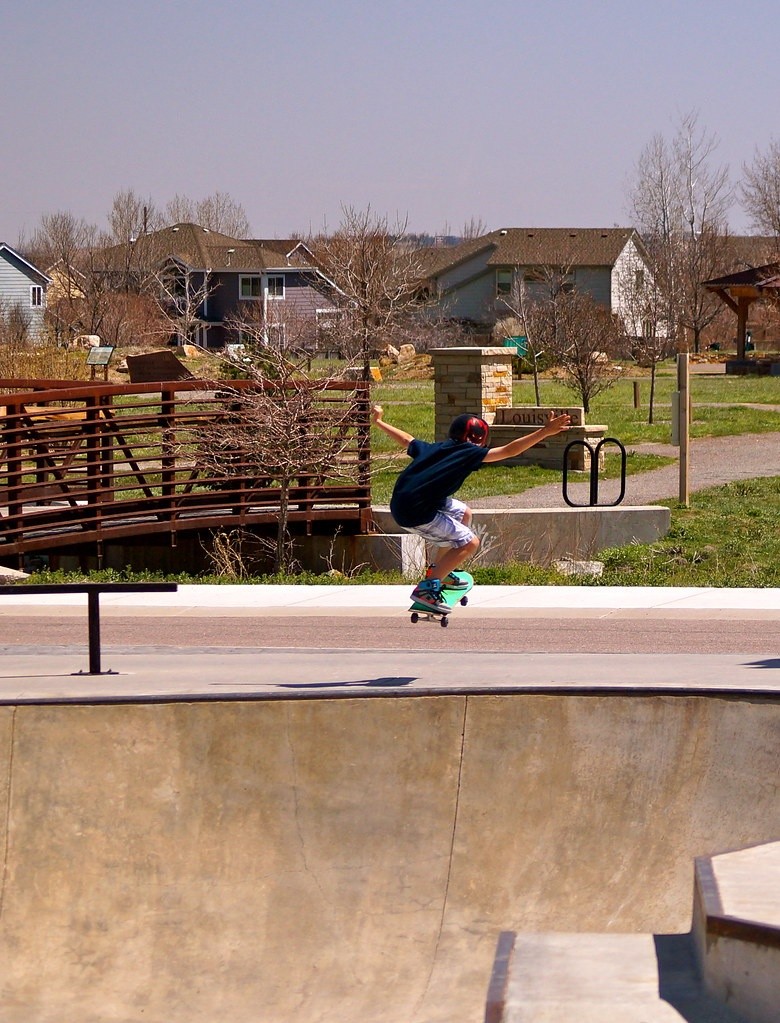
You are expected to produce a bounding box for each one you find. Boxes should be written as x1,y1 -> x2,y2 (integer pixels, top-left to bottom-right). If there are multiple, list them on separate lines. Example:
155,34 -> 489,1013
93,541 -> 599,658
425,563 -> 469,589
410,579 -> 452,614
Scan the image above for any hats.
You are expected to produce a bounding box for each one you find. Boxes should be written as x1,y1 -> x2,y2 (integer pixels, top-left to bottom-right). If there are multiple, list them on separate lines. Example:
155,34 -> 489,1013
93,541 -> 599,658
448,415 -> 491,448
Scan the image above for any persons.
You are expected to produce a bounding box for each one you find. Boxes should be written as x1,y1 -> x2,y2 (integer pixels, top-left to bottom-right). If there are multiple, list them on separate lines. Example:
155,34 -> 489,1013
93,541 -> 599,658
371,404 -> 570,615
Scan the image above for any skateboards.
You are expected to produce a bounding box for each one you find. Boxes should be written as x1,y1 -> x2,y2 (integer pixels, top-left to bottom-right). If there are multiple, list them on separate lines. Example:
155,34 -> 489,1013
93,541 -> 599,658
409,570 -> 474,628
